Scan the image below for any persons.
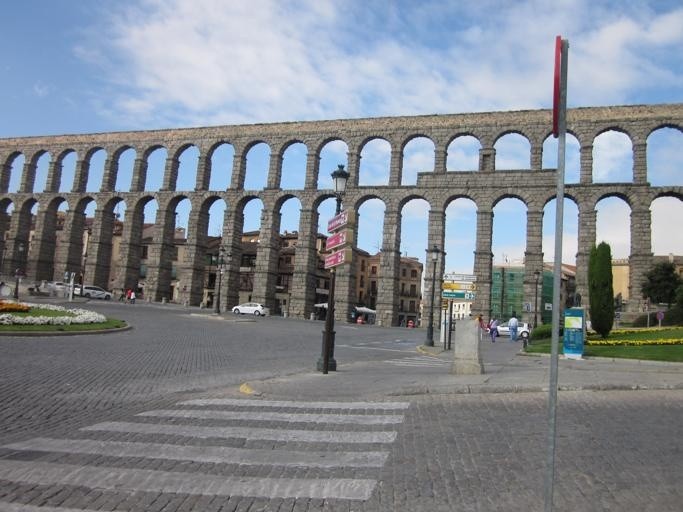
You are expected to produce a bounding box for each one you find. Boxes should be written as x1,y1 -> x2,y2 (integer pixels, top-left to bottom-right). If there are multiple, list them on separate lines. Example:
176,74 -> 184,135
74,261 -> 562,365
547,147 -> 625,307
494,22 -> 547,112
117,288 -> 124,302
477,314 -> 484,341
124,289 -> 131,303
130,291 -> 135,304
488,317 -> 497,343
507,314 -> 518,340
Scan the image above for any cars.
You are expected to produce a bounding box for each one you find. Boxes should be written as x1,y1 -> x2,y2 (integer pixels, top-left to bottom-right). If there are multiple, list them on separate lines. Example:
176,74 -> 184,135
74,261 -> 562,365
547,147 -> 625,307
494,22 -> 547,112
484,321 -> 533,339
28,279 -> 114,300
232,302 -> 270,316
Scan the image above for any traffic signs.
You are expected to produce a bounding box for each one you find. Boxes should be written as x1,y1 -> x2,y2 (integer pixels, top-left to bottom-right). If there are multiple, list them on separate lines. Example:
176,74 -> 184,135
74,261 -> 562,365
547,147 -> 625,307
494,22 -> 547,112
441,274 -> 480,299
325,210 -> 355,268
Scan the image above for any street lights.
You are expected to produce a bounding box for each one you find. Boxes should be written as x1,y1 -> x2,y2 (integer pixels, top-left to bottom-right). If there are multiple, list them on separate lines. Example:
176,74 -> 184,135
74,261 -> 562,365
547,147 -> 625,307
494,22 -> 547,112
19,243 -> 25,265
211,245 -> 232,313
533,268 -> 540,328
317,165 -> 350,370
79,251 -> 89,298
424,245 -> 440,347
